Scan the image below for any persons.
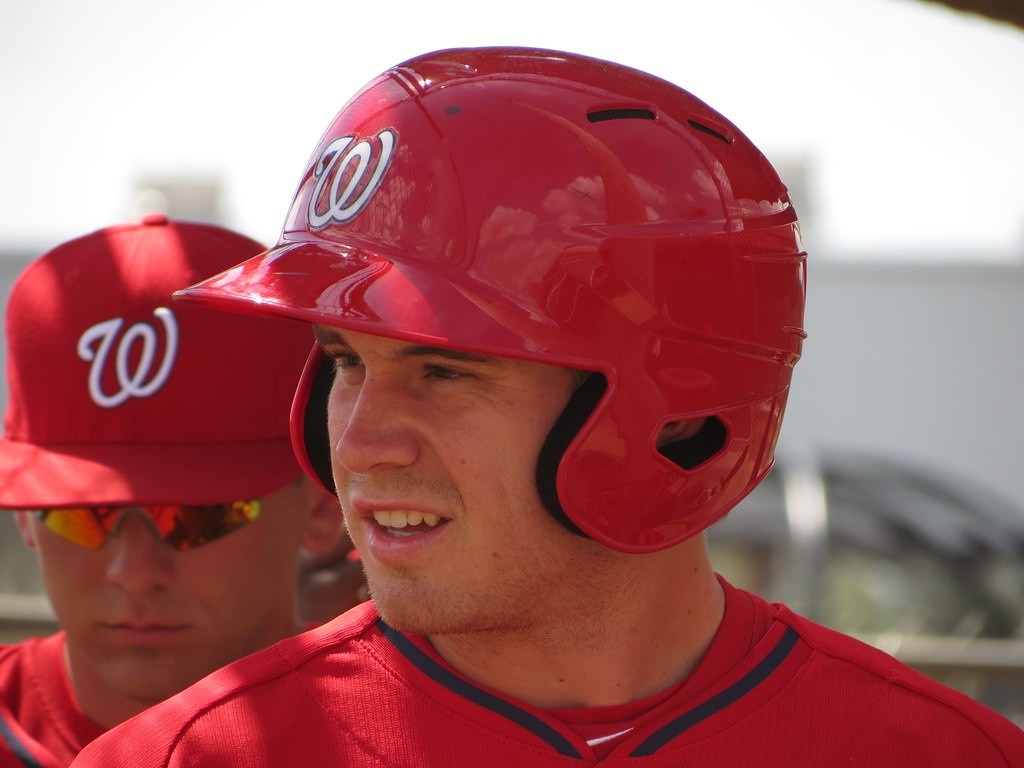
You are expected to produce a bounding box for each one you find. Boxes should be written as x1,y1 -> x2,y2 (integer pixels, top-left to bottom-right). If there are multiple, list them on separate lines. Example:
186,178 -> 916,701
0,214 -> 342,767
69,47 -> 1024,768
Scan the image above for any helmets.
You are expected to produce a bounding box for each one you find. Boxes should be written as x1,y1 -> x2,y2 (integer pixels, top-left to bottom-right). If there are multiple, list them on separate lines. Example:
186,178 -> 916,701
172,45 -> 807,554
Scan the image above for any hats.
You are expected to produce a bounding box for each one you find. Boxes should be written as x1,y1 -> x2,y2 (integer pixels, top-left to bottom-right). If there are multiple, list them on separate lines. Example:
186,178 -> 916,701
0,216 -> 319,512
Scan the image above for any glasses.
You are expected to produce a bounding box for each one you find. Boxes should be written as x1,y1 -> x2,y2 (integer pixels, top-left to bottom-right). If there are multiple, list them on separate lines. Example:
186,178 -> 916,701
30,498 -> 263,552
